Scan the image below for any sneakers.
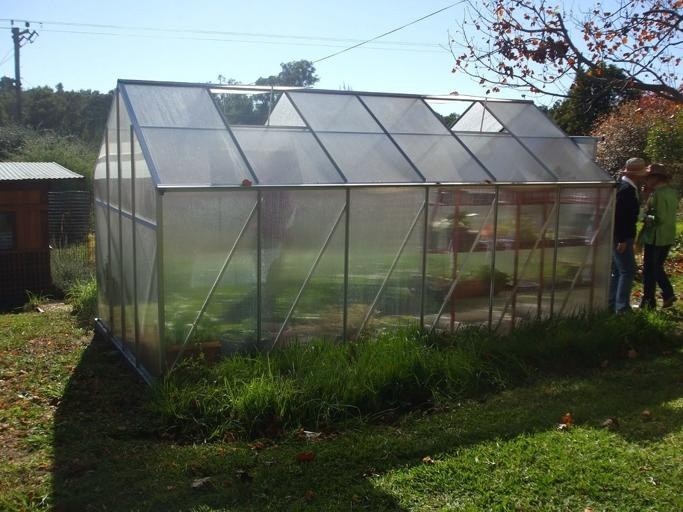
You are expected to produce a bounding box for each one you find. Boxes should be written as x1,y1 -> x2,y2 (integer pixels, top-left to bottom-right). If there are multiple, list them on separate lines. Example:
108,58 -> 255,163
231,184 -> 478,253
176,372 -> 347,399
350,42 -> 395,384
615,304 -> 633,316
661,296 -> 679,310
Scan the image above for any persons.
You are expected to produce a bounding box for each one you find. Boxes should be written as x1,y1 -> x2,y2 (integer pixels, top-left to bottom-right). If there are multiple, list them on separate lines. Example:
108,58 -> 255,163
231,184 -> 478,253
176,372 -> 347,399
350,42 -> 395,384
607,157 -> 649,315
631,163 -> 678,311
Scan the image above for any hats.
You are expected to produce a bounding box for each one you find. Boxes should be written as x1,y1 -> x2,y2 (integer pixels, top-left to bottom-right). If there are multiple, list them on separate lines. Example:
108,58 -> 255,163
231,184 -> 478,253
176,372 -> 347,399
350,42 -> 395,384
619,157 -> 651,177
649,163 -> 668,177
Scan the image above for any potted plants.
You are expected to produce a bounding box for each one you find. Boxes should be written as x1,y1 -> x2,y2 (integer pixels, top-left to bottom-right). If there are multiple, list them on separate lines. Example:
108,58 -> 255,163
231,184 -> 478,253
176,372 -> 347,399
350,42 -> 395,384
444,208 -> 471,246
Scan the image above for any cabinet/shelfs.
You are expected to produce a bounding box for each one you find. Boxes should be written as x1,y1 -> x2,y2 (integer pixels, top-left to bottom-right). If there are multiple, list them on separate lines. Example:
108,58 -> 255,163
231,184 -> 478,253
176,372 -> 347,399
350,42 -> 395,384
414,233 -> 596,301
